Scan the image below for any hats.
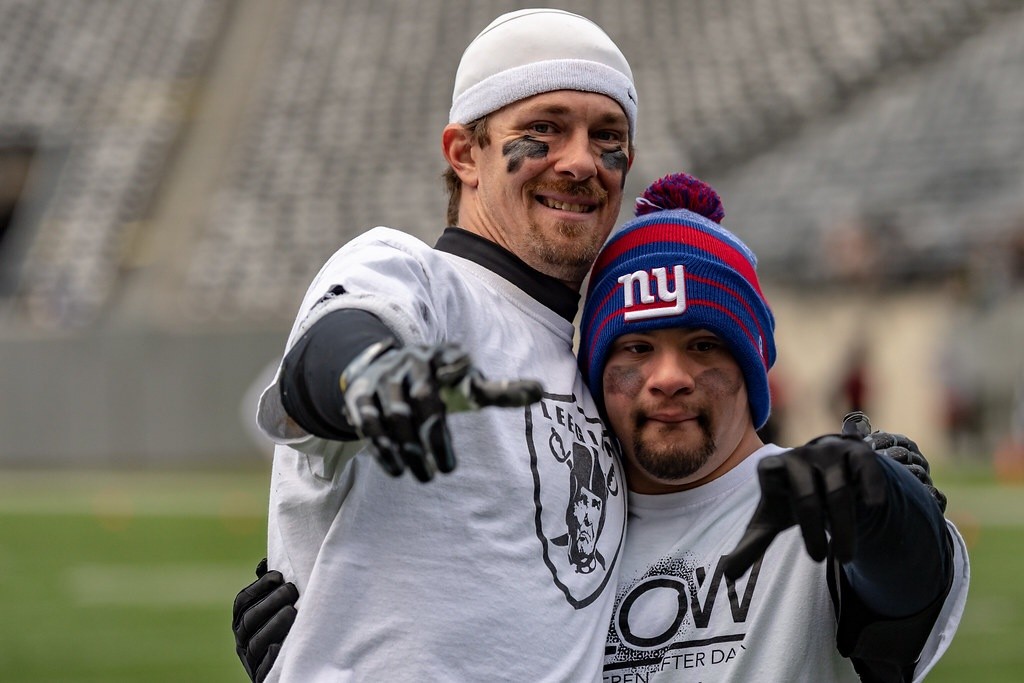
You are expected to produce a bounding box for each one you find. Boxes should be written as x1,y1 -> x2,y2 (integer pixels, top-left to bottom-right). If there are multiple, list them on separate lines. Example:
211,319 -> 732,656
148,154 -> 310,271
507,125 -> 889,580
448,8 -> 638,152
576,173 -> 777,431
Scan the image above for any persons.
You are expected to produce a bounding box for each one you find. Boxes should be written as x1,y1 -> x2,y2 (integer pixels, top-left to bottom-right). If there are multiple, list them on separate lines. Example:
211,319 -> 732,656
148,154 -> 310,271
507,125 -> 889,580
230,167 -> 972,683
254,8 -> 949,683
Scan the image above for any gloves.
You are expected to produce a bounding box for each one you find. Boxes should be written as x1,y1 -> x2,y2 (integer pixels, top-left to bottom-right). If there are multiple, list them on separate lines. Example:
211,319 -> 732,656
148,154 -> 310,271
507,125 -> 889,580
231,558 -> 299,683
841,410 -> 946,514
722,441 -> 889,581
339,334 -> 542,485
